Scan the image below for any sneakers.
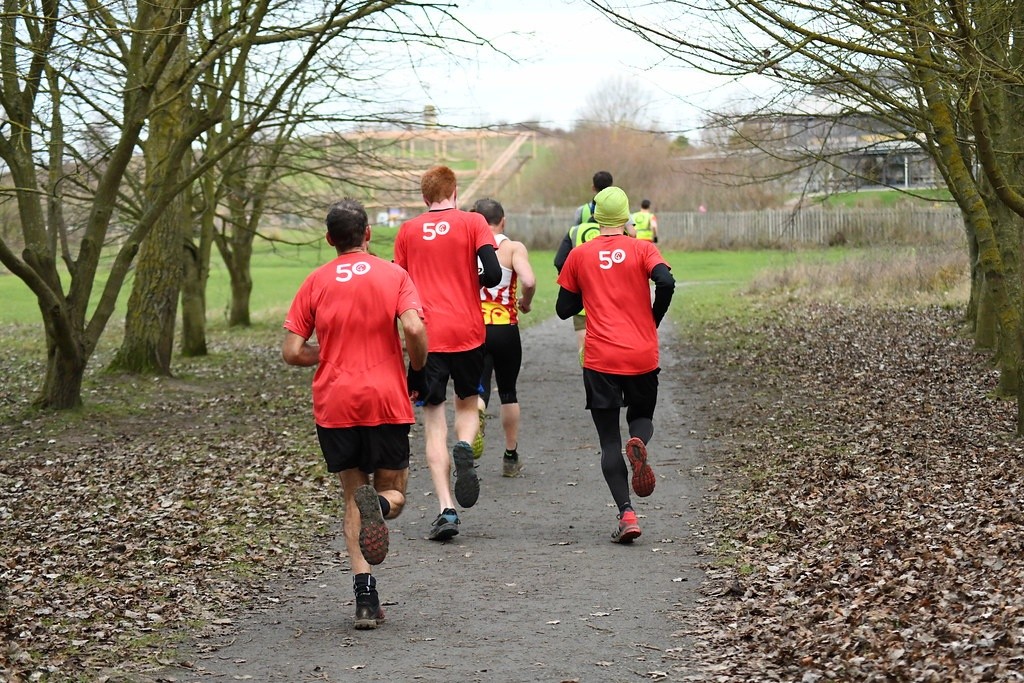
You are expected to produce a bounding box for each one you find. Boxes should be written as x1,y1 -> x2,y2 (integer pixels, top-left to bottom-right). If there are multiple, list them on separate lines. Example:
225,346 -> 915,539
471,431 -> 484,459
503,460 -> 520,476
430,514 -> 460,539
354,484 -> 390,565
610,521 -> 641,543
625,439 -> 656,497
355,598 -> 387,628
453,441 -> 481,509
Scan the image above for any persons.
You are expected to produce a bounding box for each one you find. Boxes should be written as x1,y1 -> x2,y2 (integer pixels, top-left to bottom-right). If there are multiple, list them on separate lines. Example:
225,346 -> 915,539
457,199 -> 539,476
555,186 -> 677,547
552,214 -> 605,367
390,163 -> 505,537
279,197 -> 429,631
630,199 -> 661,244
574,170 -> 615,222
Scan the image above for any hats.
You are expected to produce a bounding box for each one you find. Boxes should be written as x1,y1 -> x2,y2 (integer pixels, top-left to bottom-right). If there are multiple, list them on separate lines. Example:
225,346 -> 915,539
593,186 -> 629,227
